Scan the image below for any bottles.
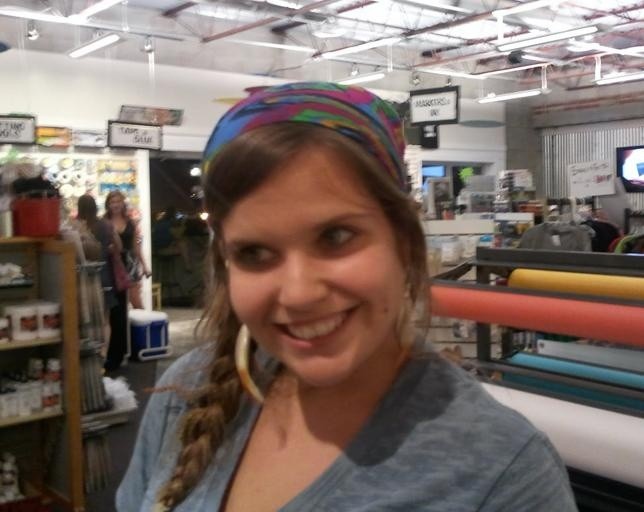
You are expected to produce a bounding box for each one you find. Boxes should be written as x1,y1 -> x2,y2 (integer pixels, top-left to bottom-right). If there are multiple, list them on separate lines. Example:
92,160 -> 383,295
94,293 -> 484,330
0,301 -> 62,503
440,235 -> 492,267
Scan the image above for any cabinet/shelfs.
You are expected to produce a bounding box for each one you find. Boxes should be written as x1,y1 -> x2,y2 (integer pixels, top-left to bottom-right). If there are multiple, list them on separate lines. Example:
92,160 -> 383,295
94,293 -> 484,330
0,234 -> 86,512
424,172 -> 543,219
419,209 -> 537,359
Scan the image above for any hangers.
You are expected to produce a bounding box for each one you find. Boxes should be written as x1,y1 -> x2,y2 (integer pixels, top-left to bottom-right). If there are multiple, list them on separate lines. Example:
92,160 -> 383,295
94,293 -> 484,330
622,209 -> 644,237
544,192 -> 595,219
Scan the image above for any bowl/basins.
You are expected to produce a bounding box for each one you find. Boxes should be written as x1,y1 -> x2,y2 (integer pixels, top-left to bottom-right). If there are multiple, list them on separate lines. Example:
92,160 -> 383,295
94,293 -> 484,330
12,199 -> 61,238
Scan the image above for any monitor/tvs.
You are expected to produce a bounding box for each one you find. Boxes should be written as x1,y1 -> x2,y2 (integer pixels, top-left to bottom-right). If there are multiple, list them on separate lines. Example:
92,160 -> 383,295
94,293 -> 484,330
617,145 -> 644,193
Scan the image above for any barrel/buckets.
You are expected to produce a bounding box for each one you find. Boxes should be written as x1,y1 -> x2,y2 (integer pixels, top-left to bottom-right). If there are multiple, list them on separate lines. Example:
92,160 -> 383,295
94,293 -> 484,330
11,189 -> 61,234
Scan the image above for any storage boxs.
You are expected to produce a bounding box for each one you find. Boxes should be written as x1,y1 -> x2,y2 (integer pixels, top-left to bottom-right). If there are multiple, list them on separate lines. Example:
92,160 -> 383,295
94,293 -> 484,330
129,307 -> 173,363
12,174 -> 61,237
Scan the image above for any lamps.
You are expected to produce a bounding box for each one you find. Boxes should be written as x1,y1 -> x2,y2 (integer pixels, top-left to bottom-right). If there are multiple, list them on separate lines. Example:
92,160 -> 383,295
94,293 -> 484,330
139,35 -> 153,53
27,21 -> 40,40
65,0 -> 129,59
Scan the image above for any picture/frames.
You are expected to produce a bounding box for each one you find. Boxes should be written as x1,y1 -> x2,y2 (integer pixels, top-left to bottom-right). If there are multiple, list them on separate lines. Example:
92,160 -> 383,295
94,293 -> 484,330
108,119 -> 162,151
0,114 -> 36,144
34,126 -> 72,146
74,129 -> 108,147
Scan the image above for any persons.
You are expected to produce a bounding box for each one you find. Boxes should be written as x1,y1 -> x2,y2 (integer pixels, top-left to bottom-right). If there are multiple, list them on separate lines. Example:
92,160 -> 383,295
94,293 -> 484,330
100,190 -> 146,367
110,81 -> 580,512
72,195 -> 123,374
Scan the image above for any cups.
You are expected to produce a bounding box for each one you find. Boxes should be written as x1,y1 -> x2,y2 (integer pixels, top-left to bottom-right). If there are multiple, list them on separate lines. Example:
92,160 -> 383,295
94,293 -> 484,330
1,211 -> 15,238
494,223 -> 528,236
